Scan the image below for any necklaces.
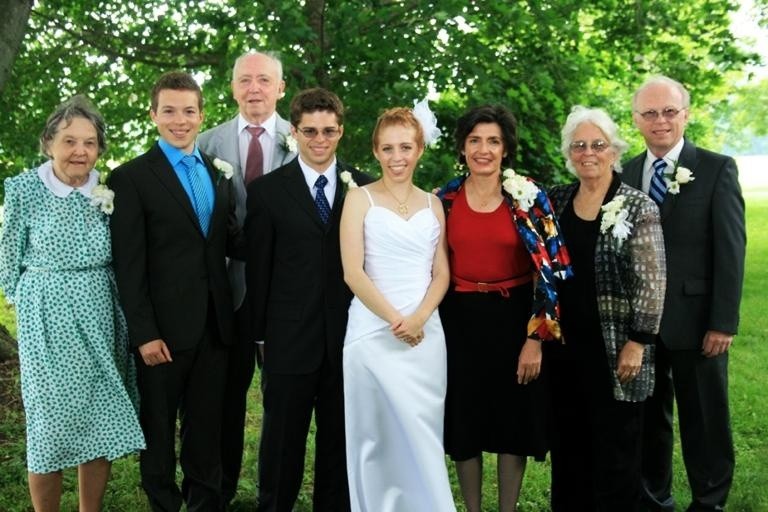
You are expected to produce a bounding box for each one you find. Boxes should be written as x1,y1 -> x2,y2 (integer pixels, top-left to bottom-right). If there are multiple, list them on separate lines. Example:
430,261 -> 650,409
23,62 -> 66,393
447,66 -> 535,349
468,177 -> 502,207
382,178 -> 413,215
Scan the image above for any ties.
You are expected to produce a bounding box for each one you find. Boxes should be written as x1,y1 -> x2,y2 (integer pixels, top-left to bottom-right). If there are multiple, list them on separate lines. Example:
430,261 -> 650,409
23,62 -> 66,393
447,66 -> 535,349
181,157 -> 211,237
648,159 -> 667,205
244,126 -> 265,184
313,175 -> 331,223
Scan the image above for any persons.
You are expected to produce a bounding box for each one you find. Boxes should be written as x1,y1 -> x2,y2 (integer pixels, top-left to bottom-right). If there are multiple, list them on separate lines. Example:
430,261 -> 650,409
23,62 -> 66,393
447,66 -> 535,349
339,108 -> 457,512
614,77 -> 747,512
437,104 -> 574,512
138,51 -> 298,506
247,89 -> 379,512
0,94 -> 146,512
106,73 -> 245,512
431,106 -> 666,512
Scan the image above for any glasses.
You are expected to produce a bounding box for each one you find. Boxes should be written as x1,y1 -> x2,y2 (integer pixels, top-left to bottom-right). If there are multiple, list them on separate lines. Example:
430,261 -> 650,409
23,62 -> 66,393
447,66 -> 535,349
569,139 -> 607,153
635,105 -> 683,121
297,126 -> 340,139
